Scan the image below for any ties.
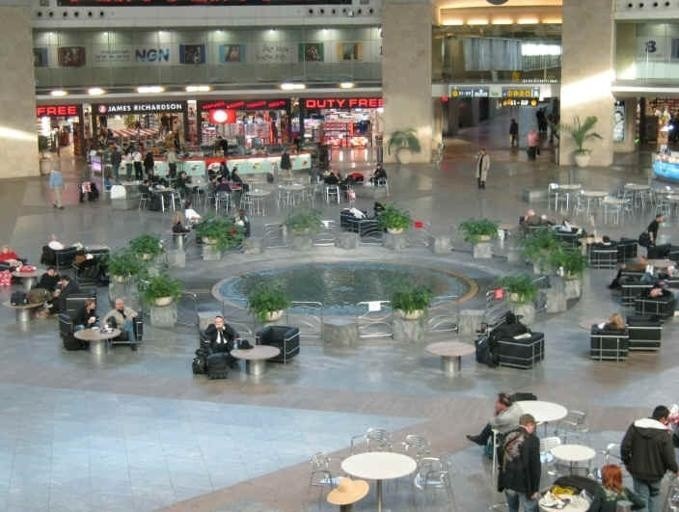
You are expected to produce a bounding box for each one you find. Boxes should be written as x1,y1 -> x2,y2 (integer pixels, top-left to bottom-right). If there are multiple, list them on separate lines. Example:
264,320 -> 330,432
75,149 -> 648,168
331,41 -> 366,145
220,330 -> 224,344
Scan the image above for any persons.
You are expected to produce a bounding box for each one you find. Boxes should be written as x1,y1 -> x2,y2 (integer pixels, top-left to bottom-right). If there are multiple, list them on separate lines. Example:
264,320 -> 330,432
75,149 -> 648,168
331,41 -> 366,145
465,393 -> 512,446
280,152 -> 388,201
585,464 -> 646,512
494,311 -> 527,342
110,111 -> 252,244
475,148 -> 490,189
102,298 -> 138,351
497,414 -> 541,512
509,107 -> 548,161
49,170 -> 65,209
620,405 -> 679,512
73,299 -> 100,331
0,232 -> 100,319
205,316 -> 235,354
520,209 -> 679,336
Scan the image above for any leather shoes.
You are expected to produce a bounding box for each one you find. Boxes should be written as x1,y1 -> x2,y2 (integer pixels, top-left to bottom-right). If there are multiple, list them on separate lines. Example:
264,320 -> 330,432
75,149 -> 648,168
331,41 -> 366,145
467,435 -> 483,445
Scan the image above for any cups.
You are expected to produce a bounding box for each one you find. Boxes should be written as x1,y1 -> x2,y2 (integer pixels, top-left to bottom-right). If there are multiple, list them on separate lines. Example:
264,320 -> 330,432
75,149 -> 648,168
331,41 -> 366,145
107,328 -> 113,334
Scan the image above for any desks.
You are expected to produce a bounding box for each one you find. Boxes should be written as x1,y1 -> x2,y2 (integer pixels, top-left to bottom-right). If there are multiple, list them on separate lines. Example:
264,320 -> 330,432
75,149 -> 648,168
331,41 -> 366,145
230,345 -> 281,376
425,341 -> 477,374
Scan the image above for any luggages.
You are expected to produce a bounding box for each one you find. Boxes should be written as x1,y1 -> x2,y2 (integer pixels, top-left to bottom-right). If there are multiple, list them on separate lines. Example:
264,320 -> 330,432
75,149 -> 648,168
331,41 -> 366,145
476,322 -> 490,362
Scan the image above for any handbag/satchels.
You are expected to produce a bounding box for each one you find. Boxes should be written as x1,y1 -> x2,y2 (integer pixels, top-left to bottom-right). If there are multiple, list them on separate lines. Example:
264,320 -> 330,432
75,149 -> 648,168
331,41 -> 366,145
497,468 -> 504,491
639,233 -> 651,246
30,288 -> 47,303
11,290 -> 29,305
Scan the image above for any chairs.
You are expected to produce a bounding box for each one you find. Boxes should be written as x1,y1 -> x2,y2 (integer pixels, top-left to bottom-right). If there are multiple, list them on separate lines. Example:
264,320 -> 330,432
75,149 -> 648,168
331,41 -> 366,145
255,325 -> 300,365
138,176 -> 390,246
200,330 -> 242,366
1,241 -> 145,354
497,183 -> 679,370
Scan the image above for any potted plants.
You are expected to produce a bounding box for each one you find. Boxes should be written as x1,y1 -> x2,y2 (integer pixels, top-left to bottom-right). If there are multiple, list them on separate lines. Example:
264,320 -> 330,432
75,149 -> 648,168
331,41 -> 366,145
379,200 -> 413,235
459,216 -> 502,260
388,127 -> 422,166
388,283 -> 436,321
245,279 -> 291,326
130,233 -> 165,264
139,273 -> 187,308
554,116 -> 604,168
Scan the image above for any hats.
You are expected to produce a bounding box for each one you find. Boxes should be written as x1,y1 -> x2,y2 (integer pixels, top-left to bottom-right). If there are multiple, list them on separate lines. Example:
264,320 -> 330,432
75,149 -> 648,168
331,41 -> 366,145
499,392 -> 511,406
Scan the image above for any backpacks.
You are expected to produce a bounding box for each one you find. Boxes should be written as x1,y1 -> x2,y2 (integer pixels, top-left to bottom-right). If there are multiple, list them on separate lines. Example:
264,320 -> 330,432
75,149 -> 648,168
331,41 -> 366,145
207,353 -> 226,379
192,349 -> 206,374
